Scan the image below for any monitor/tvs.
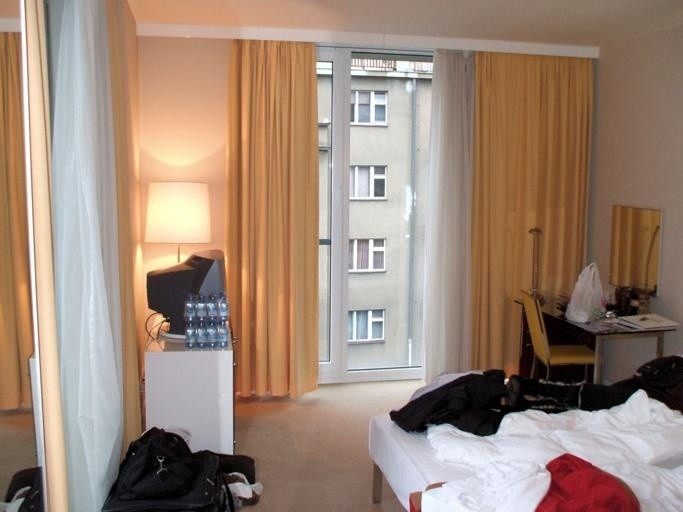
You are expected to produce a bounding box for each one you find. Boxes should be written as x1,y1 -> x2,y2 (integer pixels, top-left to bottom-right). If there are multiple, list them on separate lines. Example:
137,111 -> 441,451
146,249 -> 226,338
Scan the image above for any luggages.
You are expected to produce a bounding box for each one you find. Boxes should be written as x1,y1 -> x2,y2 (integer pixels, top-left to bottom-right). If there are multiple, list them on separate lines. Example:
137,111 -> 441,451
103,450 -> 225,512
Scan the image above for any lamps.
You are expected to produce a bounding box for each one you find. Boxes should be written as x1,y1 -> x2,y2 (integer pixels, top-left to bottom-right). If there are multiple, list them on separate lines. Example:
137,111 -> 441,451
144,181 -> 212,265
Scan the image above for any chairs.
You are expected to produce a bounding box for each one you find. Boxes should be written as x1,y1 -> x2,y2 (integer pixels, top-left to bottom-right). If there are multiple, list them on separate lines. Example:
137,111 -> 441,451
519,288 -> 605,384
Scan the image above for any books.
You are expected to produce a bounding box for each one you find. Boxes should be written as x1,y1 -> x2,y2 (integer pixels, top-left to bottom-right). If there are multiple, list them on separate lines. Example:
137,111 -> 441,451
618,315 -> 682,332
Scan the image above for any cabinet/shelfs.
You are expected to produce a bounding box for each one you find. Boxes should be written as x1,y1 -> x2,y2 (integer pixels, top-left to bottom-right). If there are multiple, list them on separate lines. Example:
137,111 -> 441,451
144,314 -> 236,455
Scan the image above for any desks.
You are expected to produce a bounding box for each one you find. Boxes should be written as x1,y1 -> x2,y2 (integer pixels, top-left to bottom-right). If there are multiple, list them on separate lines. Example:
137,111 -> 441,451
512,297 -> 678,384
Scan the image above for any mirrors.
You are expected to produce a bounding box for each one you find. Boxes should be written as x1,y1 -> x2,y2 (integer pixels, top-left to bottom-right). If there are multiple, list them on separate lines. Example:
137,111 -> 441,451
609,204 -> 663,298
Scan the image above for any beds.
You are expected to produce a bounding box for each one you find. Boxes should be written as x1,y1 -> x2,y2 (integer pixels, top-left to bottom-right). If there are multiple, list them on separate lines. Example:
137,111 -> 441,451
370,406 -> 683,512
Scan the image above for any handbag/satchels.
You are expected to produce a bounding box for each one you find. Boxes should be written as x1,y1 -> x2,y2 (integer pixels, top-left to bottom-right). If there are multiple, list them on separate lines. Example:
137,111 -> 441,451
218,454 -> 255,483
5,467 -> 43,512
116,428 -> 195,499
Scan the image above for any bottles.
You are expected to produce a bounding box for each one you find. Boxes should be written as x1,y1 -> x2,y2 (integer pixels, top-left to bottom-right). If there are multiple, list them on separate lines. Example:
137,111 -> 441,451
182,291 -> 230,350
